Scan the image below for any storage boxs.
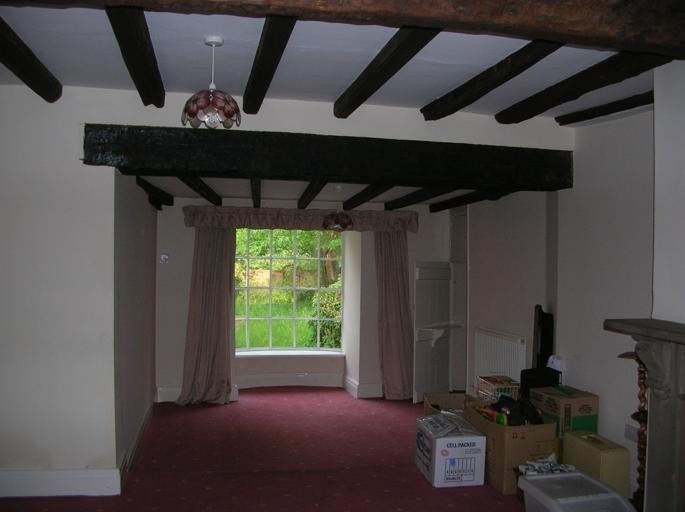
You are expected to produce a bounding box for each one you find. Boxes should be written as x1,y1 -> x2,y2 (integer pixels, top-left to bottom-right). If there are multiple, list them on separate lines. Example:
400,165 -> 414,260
530,385 -> 599,439
423,391 -> 477,419
466,400 -> 560,495
414,411 -> 486,488
518,470 -> 639,512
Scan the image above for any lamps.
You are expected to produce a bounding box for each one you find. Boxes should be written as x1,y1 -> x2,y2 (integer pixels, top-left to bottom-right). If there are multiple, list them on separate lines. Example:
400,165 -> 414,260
321,185 -> 353,232
181,31 -> 243,130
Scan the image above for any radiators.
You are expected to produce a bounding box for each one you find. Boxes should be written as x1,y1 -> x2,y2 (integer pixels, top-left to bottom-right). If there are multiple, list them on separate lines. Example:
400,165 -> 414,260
475,328 -> 526,383
